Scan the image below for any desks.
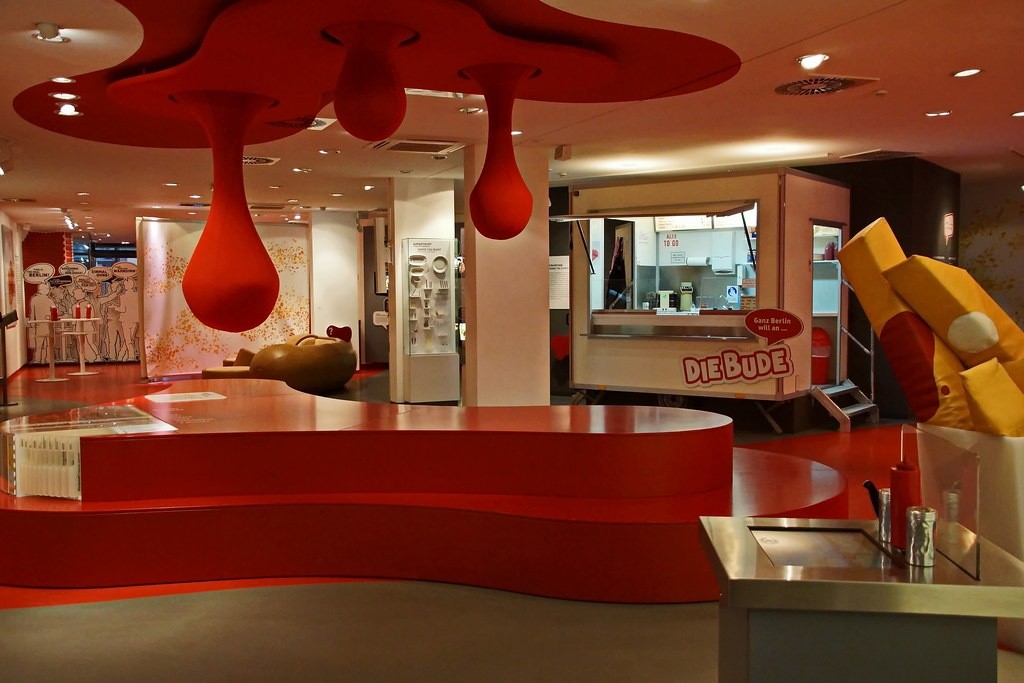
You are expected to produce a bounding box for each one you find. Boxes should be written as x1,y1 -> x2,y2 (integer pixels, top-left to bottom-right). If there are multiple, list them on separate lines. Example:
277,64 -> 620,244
697,511 -> 1024,683
29,317 -> 71,382
59,318 -> 102,376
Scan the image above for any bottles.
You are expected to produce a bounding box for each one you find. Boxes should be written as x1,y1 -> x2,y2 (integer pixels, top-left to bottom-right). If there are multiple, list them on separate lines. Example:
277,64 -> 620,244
825,241 -> 837,260
905,506 -> 938,566
642,302 -> 649,310
942,490 -> 961,544
890,423 -> 923,549
51,306 -> 57,321
76,305 -> 81,319
86,304 -> 91,319
747,249 -> 756,263
459,322 -> 467,341
878,487 -> 890,544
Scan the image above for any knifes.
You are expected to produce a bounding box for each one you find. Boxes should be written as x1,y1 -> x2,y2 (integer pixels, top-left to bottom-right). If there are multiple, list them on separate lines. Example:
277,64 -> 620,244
862,479 -> 879,518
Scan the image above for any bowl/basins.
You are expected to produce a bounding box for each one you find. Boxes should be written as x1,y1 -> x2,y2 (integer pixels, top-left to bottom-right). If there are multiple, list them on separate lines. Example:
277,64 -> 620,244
813,254 -> 825,261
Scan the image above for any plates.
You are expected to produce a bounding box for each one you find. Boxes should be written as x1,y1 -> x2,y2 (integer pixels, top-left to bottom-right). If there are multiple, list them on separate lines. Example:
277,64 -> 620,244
432,255 -> 449,274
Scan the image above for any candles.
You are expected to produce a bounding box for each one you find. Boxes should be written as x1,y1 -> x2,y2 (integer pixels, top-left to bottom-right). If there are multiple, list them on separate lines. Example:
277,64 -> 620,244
51,308 -> 58,321
76,305 -> 81,319
86,304 -> 91,318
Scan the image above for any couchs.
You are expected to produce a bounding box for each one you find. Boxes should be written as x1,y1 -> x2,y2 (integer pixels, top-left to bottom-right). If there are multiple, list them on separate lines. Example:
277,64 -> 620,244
200,333 -> 357,394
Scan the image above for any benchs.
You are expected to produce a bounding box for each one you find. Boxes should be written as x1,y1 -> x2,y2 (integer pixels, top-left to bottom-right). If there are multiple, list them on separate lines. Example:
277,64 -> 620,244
2,379 -> 849,607
